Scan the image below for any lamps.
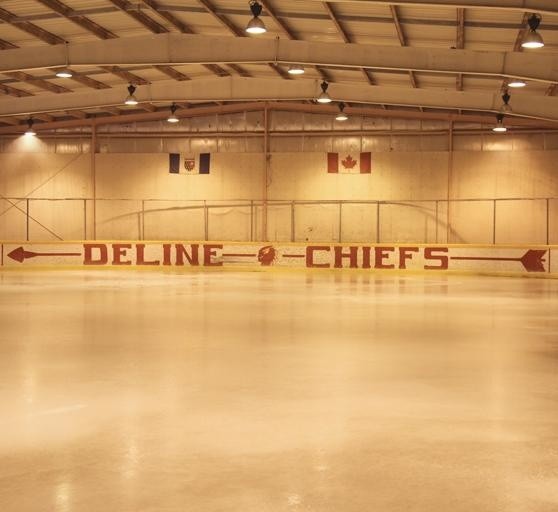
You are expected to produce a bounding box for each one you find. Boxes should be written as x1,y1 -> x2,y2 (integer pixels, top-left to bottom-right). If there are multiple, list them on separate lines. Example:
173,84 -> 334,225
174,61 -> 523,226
165,104 -> 179,122
491,77 -> 527,133
245,2 -> 268,35
124,82 -> 138,105
287,63 -> 348,121
520,12 -> 545,48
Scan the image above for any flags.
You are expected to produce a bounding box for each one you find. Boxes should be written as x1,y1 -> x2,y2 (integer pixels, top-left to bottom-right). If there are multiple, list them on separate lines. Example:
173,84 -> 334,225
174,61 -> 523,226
168,151 -> 210,176
326,149 -> 372,176
